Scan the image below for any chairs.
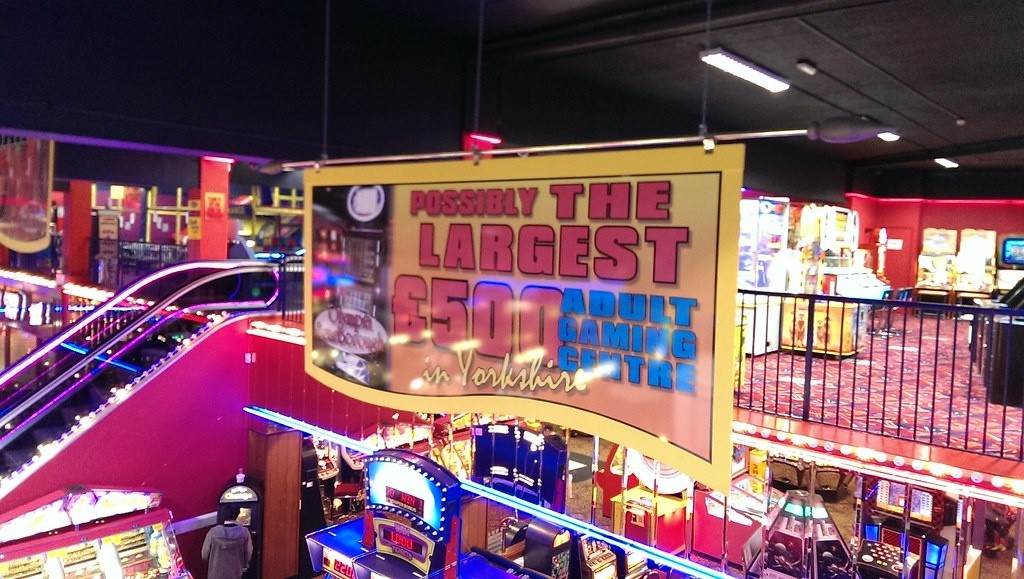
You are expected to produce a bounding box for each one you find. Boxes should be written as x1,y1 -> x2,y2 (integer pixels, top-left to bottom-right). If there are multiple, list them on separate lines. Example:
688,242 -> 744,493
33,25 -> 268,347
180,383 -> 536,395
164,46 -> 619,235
868,290 -> 899,337
882,287 -> 913,335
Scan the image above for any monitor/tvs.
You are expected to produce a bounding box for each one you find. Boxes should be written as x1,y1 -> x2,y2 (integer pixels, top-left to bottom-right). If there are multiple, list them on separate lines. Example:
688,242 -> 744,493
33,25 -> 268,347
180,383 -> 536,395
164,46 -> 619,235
999,234 -> 1024,270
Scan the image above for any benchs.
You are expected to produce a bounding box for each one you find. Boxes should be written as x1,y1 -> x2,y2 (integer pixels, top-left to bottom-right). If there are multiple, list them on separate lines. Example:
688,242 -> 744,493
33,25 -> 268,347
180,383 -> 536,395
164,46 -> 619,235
914,290 -> 948,320
957,292 -> 990,305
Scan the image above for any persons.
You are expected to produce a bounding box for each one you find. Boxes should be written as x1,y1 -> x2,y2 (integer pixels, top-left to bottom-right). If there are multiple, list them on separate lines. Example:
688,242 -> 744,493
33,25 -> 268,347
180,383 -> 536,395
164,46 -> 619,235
544,424 -> 566,450
985,501 -> 1017,558
201,504 -> 253,579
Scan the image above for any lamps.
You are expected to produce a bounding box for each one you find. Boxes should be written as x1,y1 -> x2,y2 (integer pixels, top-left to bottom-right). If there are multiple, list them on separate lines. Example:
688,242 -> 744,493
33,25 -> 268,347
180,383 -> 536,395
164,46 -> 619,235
700,47 -> 791,93
473,0 -> 485,165
699,0 -> 715,154
